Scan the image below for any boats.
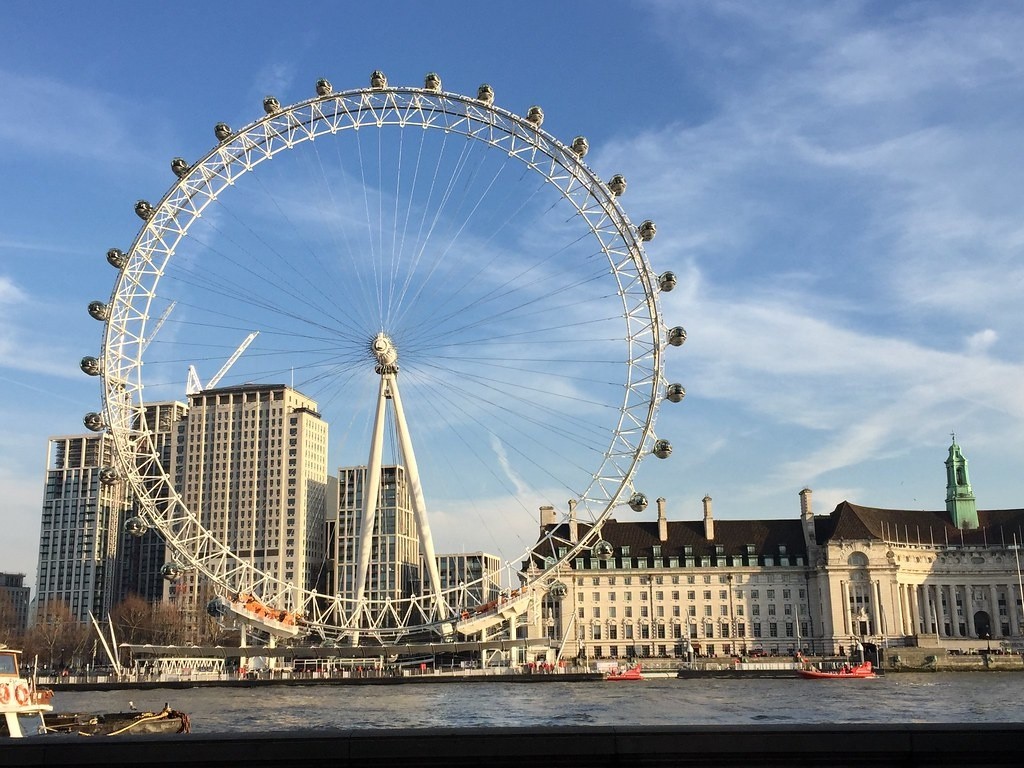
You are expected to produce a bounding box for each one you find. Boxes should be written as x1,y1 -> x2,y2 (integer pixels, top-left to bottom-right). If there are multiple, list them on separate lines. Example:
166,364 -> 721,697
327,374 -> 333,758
798,661 -> 876,678
41,703 -> 190,736
0,644 -> 79,738
607,664 -> 643,680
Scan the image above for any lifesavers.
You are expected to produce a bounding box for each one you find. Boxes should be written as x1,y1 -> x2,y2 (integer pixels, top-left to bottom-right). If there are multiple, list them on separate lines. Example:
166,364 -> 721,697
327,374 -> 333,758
15,684 -> 29,705
735,659 -> 740,663
0,683 -> 11,704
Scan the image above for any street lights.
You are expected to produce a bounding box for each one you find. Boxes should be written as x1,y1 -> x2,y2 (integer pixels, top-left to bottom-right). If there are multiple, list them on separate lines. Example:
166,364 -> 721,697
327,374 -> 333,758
986,632 -> 992,654
